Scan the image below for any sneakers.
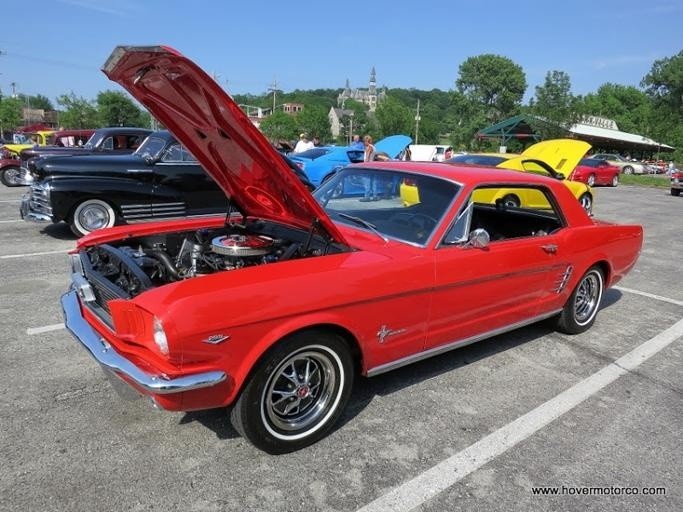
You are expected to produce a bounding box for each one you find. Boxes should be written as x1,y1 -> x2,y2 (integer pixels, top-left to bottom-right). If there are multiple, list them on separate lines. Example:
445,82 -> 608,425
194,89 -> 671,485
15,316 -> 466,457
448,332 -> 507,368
360,196 -> 380,202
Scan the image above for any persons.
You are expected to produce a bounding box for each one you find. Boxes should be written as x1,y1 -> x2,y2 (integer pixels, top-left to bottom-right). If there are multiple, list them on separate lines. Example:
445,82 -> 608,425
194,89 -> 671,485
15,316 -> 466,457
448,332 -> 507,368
59,126 -> 75,147
444,146 -> 453,160
294,133 -> 324,153
668,160 -> 674,176
349,135 -> 365,149
360,134 -> 377,202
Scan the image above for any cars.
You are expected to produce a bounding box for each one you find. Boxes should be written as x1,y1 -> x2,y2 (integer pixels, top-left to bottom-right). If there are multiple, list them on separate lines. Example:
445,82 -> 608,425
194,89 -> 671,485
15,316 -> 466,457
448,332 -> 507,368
669,164 -> 683,196
568,158 -> 621,188
288,134 -> 412,201
0,125 -> 154,189
18,128 -> 316,238
60,42 -> 644,455
399,139 -> 596,218
588,153 -> 671,176
